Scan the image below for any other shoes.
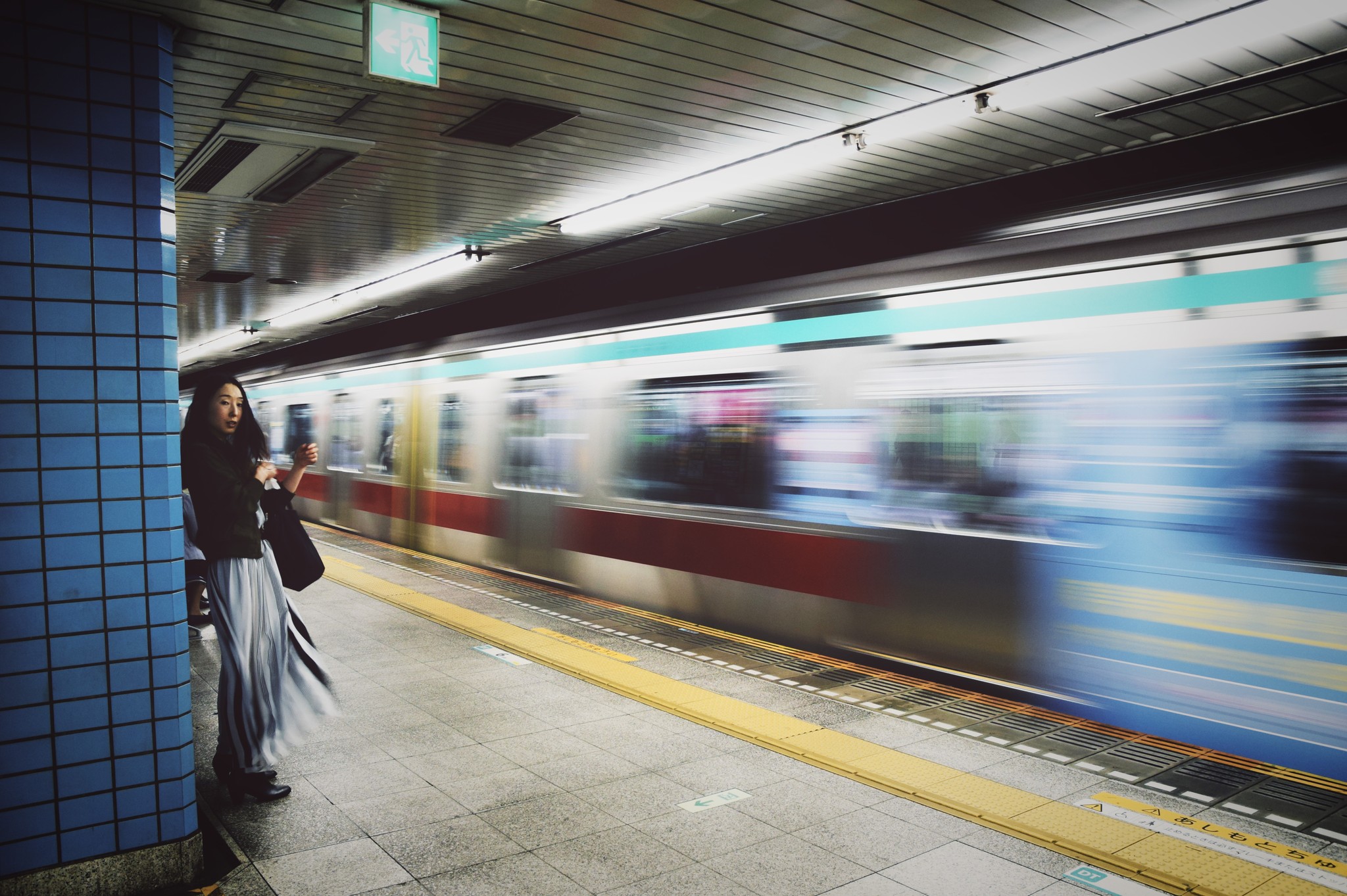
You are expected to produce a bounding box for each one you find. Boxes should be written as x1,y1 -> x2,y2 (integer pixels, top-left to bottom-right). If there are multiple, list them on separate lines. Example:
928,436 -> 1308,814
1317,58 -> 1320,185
198,596 -> 209,609
189,611 -> 211,626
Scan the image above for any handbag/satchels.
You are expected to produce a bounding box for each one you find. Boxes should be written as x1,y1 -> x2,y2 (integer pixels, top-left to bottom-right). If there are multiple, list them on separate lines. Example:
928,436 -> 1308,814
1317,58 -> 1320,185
260,481 -> 325,592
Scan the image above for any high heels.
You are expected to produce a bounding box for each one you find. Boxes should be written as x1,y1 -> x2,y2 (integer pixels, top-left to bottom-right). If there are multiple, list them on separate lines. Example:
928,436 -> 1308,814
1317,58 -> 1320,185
213,760 -> 277,785
226,763 -> 292,805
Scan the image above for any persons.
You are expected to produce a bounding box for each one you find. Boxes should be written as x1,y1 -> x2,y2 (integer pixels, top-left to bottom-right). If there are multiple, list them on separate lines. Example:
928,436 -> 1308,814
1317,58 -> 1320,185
181,490 -> 215,623
181,372 -> 319,803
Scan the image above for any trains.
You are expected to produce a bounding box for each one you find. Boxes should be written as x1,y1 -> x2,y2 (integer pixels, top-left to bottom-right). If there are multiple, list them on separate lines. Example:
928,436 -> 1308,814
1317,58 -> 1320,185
178,231 -> 1347,790
